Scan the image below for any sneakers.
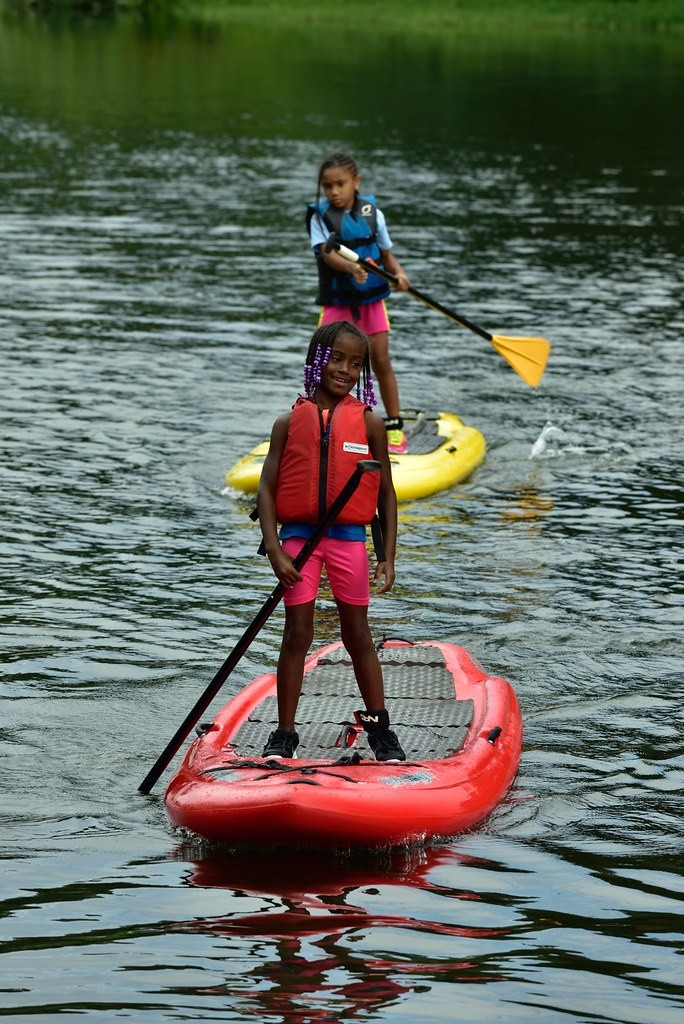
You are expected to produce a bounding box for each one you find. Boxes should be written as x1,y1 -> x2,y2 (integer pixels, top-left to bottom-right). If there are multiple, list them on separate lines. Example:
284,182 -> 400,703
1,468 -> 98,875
261,730 -> 300,761
367,726 -> 407,764
386,430 -> 408,455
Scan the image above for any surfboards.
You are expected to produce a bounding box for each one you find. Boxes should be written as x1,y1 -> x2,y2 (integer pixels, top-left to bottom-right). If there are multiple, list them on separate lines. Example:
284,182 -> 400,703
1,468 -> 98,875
226,405 -> 490,507
165,638 -> 526,841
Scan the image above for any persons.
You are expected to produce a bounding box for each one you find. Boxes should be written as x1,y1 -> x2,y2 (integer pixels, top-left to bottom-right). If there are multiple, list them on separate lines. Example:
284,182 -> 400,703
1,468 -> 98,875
305,154 -> 409,454
258,321 -> 407,763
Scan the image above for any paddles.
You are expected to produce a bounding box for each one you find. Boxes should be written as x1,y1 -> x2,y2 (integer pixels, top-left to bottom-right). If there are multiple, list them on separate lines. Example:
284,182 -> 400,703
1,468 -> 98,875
137,459 -> 384,796
324,229 -> 551,392
